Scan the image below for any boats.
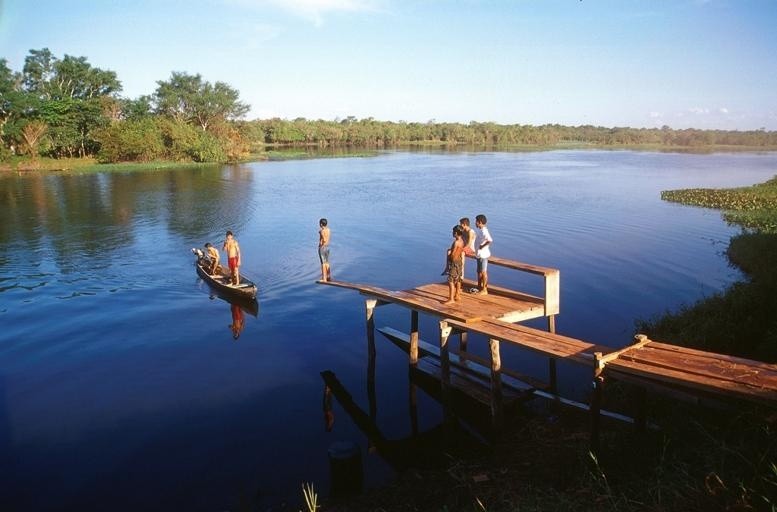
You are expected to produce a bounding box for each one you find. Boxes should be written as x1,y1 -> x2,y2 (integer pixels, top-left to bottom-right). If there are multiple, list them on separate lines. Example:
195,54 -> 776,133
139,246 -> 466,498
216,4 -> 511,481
193,278 -> 258,318
195,255 -> 258,300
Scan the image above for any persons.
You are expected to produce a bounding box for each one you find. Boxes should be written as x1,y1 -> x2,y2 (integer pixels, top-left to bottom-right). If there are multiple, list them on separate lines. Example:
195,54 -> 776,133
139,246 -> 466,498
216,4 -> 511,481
209,294 -> 215,299
474,215 -> 492,295
441,218 -> 477,275
323,385 -> 334,432
204,243 -> 220,275
318,219 -> 331,282
229,304 -> 245,340
442,225 -> 464,305
223,231 -> 241,285
